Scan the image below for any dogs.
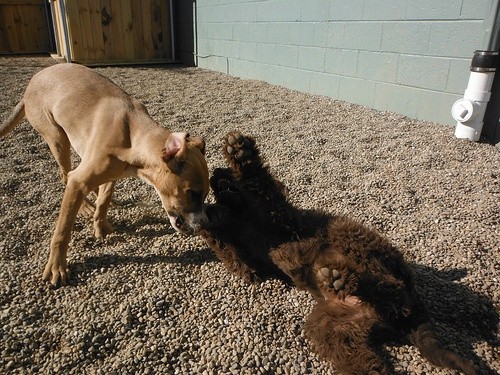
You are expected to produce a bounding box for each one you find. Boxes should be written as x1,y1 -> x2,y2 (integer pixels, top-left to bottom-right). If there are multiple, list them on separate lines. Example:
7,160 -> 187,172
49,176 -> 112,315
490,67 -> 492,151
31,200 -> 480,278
197,129 -> 483,375
1,62 -> 211,288
